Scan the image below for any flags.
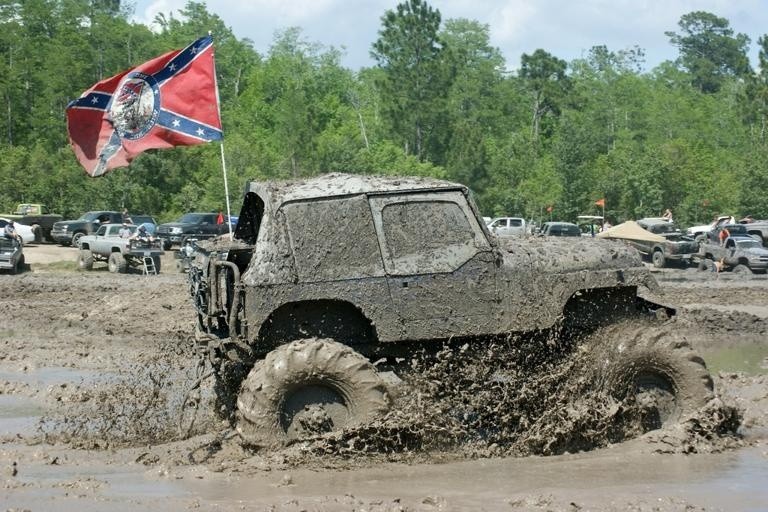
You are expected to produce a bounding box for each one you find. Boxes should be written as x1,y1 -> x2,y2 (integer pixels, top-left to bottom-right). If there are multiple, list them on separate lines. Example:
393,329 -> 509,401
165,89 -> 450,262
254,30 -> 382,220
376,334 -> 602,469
65,32 -> 226,178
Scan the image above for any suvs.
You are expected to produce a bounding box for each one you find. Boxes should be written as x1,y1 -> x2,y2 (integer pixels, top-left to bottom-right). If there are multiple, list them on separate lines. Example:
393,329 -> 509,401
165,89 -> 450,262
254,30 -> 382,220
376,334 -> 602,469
189,172 -> 715,463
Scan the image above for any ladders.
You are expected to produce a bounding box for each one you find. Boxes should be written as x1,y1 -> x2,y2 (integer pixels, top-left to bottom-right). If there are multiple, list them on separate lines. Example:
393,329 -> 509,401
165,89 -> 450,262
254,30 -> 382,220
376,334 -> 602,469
142,257 -> 158,276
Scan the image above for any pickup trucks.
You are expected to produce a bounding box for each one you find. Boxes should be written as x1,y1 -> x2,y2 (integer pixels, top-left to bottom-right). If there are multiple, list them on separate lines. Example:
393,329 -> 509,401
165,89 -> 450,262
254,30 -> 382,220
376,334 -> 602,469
0,210 -> 247,277
478,207 -> 768,276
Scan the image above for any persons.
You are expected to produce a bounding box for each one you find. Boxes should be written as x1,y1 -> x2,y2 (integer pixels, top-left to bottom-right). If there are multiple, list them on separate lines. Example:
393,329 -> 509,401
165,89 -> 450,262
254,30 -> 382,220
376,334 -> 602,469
719,227 -> 730,247
662,208 -> 673,225
3,220 -> 21,245
497,220 -> 505,226
97,205 -> 156,243
